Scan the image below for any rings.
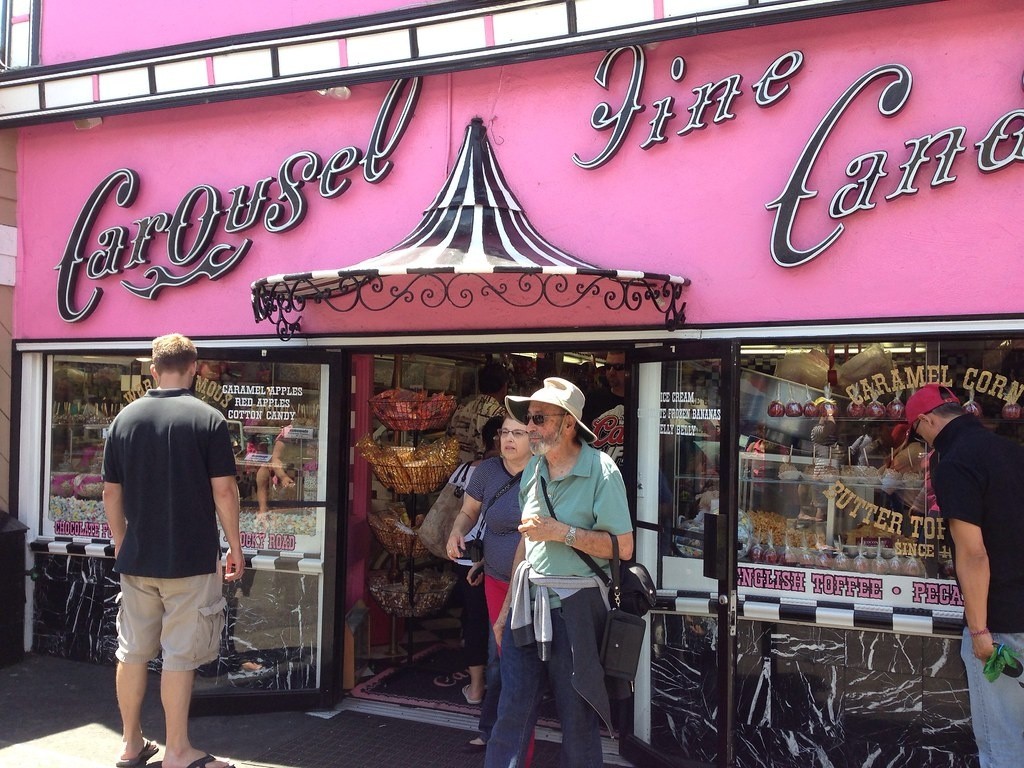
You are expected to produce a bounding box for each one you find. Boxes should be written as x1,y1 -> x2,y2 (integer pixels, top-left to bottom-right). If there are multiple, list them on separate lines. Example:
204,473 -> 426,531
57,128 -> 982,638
525,532 -> 528,537
448,550 -> 451,553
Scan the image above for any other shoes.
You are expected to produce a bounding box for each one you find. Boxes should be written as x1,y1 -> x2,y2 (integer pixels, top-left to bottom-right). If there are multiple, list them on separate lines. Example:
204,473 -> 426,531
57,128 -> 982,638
456,741 -> 486,753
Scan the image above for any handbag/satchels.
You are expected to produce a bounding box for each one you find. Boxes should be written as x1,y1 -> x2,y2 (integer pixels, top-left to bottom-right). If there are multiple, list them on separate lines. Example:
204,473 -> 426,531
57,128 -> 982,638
599,533 -> 646,680
608,561 -> 657,616
417,461 -> 473,561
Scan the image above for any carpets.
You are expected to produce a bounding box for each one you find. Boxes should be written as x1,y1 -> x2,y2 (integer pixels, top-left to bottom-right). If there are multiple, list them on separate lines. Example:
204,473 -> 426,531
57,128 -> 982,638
243,709 -> 632,768
351,642 -> 620,738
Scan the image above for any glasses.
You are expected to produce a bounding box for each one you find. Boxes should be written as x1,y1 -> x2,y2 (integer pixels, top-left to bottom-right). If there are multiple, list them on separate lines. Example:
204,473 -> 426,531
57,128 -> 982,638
604,363 -> 625,371
911,412 -> 932,441
498,428 -> 527,438
522,414 -> 569,425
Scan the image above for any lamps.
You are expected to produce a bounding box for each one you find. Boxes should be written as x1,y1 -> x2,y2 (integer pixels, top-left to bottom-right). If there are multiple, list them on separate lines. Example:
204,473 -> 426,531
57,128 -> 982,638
73,118 -> 103,129
316,86 -> 353,100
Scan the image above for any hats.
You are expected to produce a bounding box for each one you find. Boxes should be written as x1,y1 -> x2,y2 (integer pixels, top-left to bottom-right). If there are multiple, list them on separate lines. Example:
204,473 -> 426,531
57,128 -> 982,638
505,377 -> 597,444
907,384 -> 960,443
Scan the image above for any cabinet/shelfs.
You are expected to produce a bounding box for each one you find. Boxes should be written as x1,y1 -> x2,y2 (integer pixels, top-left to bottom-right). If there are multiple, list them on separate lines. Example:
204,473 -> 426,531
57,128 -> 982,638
48,352 -> 321,515
369,354 -> 488,573
670,342 -> 1024,580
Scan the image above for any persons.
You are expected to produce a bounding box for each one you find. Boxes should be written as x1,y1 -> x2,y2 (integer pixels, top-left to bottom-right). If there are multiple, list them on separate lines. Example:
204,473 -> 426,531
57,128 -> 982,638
577,348 -> 625,471
102,332 -> 246,768
254,410 -> 308,518
479,377 -> 633,768
748,399 -> 845,521
904,383 -> 1024,768
849,421 -> 950,579
445,415 -> 535,768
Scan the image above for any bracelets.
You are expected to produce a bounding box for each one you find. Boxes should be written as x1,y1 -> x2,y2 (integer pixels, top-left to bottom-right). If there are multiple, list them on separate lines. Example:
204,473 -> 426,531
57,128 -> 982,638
970,628 -> 989,637
827,414 -> 833,416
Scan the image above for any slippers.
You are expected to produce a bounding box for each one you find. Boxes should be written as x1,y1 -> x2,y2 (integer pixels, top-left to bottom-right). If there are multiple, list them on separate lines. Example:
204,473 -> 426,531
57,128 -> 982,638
185,752 -> 235,768
462,684 -> 482,704
115,738 -> 159,766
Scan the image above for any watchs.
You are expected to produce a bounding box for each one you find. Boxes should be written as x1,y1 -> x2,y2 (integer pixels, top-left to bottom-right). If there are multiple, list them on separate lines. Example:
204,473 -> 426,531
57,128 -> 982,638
565,526 -> 577,546
